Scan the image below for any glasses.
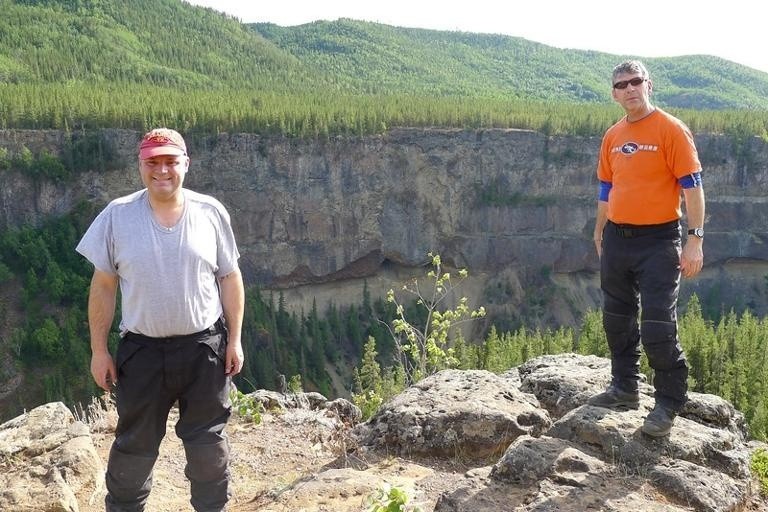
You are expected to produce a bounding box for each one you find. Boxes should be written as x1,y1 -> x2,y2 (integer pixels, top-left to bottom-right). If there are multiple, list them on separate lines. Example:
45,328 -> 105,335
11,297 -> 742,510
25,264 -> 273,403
611,77 -> 645,88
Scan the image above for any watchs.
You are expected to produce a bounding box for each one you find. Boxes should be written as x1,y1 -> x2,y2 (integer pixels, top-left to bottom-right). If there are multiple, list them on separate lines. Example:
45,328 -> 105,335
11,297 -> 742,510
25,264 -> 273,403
687,227 -> 704,238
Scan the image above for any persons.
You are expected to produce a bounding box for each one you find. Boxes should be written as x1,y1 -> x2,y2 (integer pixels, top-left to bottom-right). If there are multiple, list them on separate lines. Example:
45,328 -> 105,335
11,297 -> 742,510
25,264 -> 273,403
588,58 -> 707,439
75,127 -> 246,511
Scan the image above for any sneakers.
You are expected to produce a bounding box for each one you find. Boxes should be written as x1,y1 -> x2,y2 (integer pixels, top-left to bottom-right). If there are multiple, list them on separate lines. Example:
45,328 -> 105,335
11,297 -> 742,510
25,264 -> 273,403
589,386 -> 640,406
642,403 -> 676,436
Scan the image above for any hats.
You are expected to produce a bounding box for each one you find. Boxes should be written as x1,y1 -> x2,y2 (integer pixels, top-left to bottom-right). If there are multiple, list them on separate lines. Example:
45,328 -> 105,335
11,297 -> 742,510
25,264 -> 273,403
139,129 -> 186,159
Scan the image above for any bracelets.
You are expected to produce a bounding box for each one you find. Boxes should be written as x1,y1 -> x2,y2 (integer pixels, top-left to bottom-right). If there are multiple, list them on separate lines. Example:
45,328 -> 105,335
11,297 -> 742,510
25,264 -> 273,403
593,230 -> 603,241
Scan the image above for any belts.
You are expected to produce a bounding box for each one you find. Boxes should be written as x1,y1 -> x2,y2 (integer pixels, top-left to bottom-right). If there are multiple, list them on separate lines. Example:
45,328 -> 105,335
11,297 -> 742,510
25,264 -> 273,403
125,319 -> 222,341
606,221 -> 680,237
